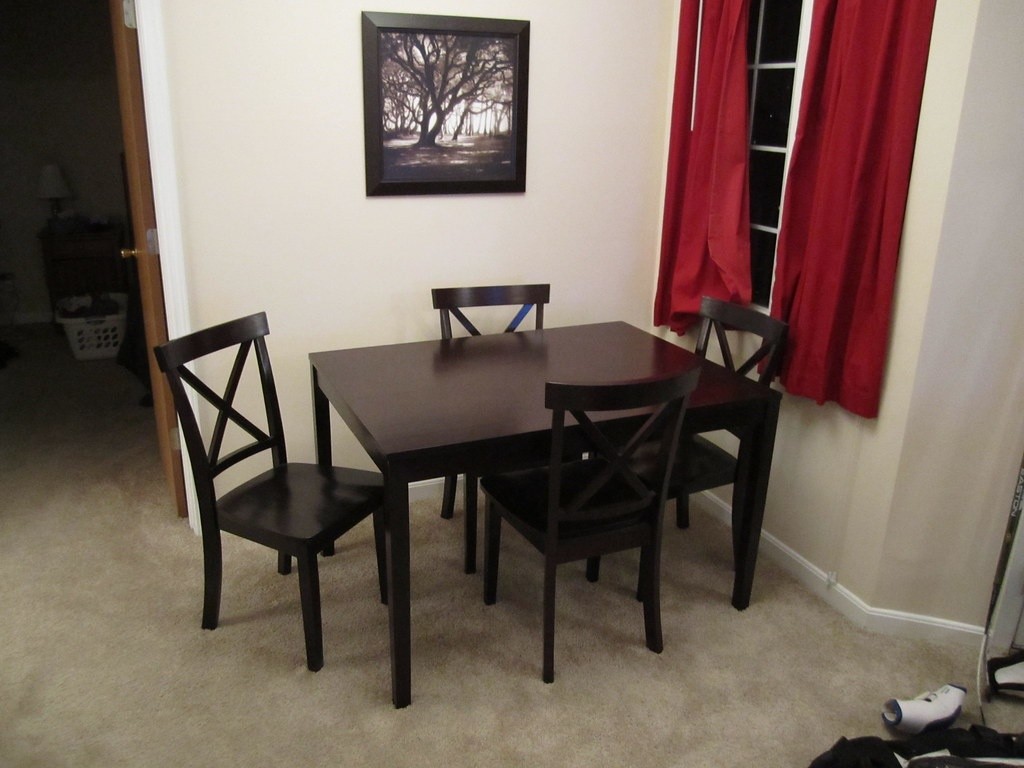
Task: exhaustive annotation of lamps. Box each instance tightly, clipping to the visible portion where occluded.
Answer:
[40,164,71,235]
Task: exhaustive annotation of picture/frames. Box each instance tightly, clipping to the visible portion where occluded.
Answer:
[362,11,529,197]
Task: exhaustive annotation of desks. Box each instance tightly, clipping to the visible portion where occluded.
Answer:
[308,320,783,709]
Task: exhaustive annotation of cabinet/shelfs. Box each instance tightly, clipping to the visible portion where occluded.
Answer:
[37,221,131,335]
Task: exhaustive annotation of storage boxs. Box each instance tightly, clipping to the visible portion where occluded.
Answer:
[55,293,128,360]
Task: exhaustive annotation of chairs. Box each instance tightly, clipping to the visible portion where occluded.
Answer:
[431,284,550,574]
[480,367,701,683]
[591,294,790,572]
[153,311,389,672]
